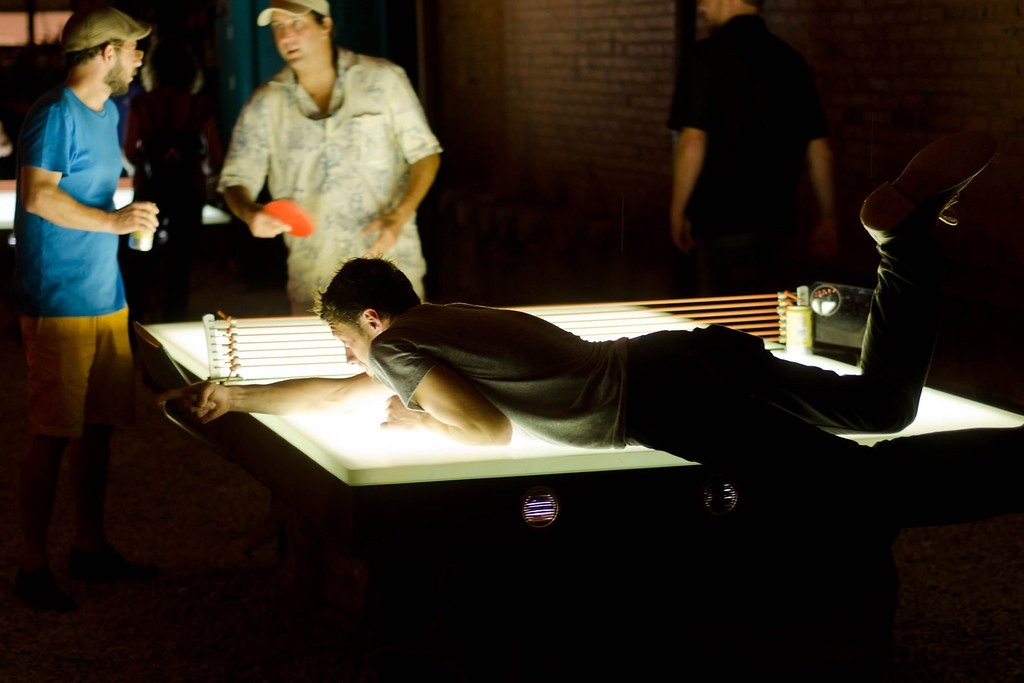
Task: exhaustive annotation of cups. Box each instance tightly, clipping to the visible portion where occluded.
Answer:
[126,225,156,250]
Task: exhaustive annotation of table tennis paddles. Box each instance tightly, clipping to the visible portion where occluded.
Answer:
[260,197,315,239]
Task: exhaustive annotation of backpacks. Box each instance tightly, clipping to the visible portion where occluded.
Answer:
[134,89,207,226]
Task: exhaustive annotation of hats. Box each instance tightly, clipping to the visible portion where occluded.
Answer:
[60,4,154,55]
[256,0,331,26]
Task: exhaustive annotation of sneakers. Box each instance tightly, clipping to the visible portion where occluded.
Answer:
[14,561,78,615]
[862,129,999,254]
[63,542,166,585]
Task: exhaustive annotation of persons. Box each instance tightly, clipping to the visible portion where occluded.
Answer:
[669,0,841,301]
[120,12,232,323]
[216,0,444,321]
[152,128,1023,534]
[11,5,162,618]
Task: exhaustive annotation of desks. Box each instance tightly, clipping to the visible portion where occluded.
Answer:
[134,302,1024,682]
